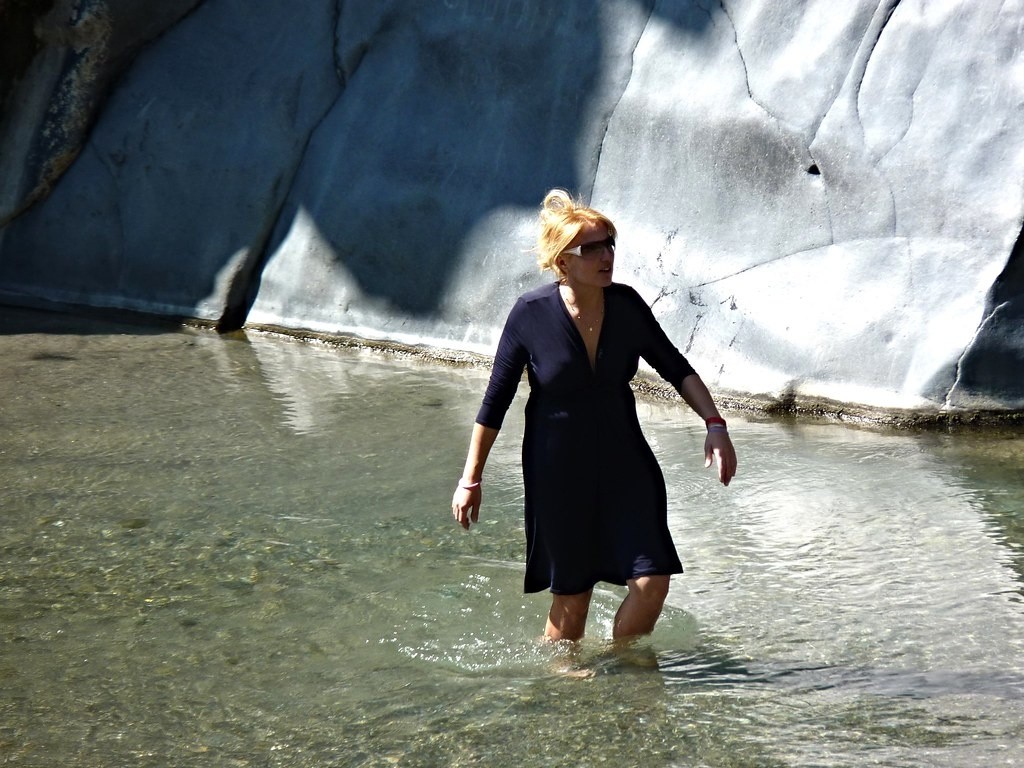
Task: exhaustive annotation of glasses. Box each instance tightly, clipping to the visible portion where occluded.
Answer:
[559,235,616,258]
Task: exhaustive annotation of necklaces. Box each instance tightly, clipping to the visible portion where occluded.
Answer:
[562,286,604,333]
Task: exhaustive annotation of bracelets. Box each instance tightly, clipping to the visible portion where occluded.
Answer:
[458,478,483,488]
[706,418,728,434]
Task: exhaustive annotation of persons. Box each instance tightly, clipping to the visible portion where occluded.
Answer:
[451,188,738,680]
